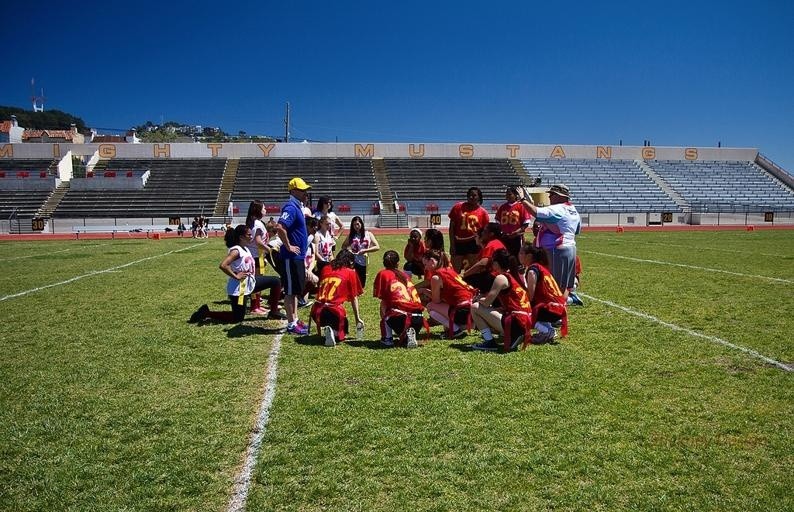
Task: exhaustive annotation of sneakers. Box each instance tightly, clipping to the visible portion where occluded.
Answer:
[285,324,307,335]
[324,326,336,346]
[296,319,309,329]
[379,324,553,352]
[569,292,583,306]
[250,296,312,319]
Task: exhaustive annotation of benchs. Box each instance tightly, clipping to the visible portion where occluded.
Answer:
[520,158,683,212]
[230,156,382,215]
[1,155,228,222]
[644,155,794,212]
[382,156,526,213]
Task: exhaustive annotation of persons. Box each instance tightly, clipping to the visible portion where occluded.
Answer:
[191,199,288,323]
[276,178,380,347]
[373,185,580,352]
[179,215,209,239]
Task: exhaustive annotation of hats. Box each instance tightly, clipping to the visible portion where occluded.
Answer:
[544,183,571,199]
[286,177,312,191]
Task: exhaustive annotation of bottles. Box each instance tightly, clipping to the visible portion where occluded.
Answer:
[356,322,364,342]
